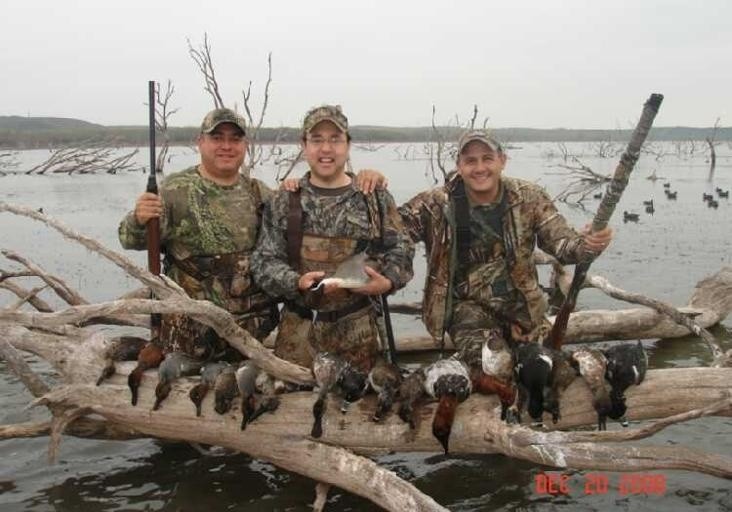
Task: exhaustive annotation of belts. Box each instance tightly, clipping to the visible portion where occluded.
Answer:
[287,300,370,321]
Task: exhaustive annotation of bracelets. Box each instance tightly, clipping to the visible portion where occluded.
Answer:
[134,211,143,228]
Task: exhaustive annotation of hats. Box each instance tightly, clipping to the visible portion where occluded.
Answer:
[201,108,246,137]
[458,131,502,153]
[305,107,348,133]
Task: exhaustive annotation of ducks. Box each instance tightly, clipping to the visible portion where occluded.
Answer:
[309,241,383,326]
[308,339,569,455]
[573,342,654,435]
[94,334,279,428]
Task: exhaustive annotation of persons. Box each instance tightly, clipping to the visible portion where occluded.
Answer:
[117,109,303,364]
[354,127,612,368]
[252,103,413,375]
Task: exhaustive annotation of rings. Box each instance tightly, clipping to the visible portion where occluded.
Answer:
[601,243,606,250]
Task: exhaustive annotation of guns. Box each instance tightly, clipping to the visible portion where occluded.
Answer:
[544,93,663,348]
[371,294,394,364]
[146,82,162,344]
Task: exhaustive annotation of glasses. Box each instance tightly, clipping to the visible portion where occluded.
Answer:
[305,137,347,148]
